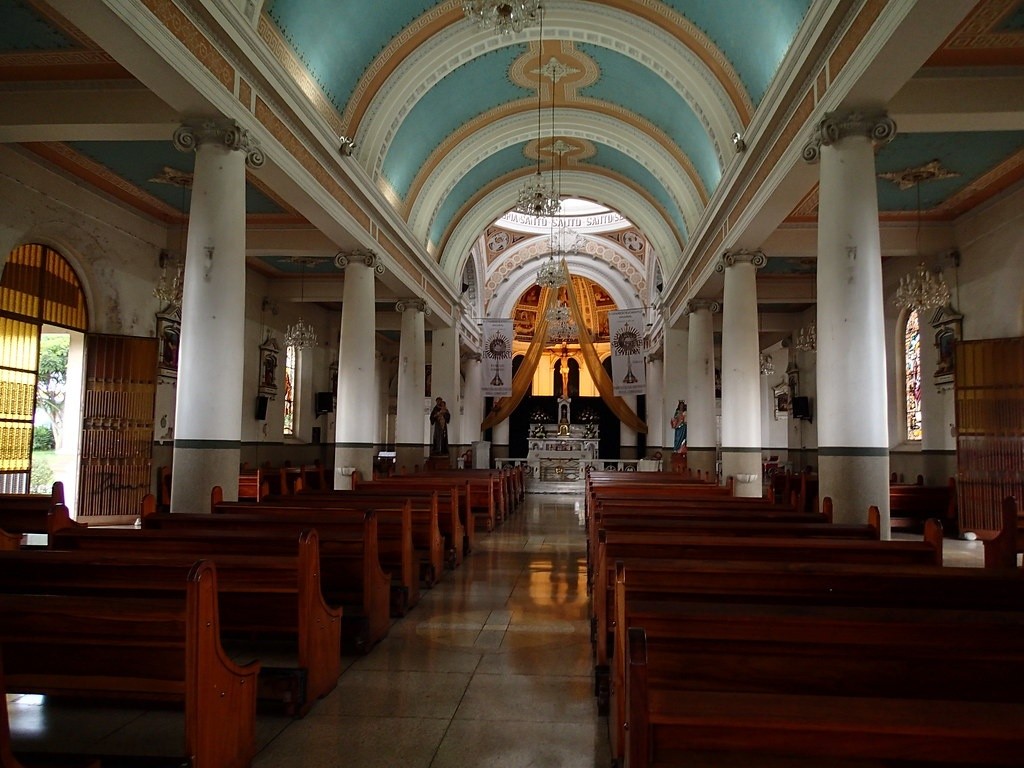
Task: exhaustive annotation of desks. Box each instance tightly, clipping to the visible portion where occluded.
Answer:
[585,464,1024,768]
[0,457,528,768]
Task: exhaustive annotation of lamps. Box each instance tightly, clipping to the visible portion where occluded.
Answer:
[464,0,607,341]
[878,160,959,314]
[147,163,195,308]
[759,299,775,376]
[282,257,319,353]
[795,264,817,353]
